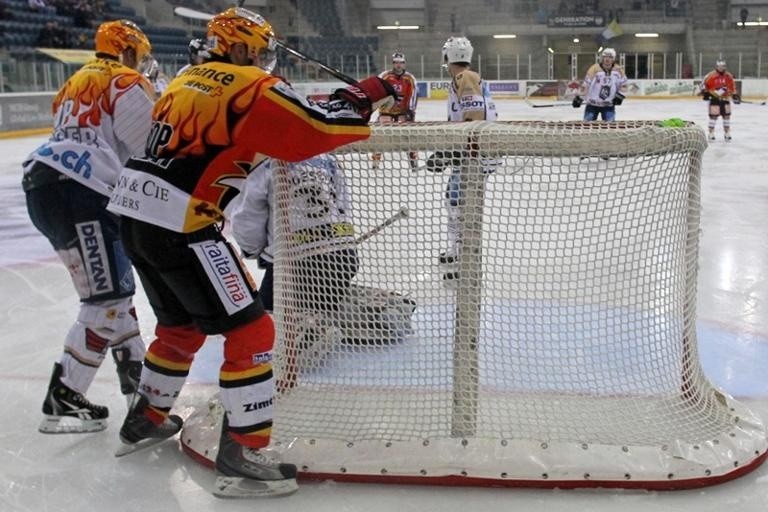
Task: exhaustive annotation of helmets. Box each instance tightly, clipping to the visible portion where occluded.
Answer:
[95,19,158,79]
[188,38,212,65]
[601,47,616,64]
[442,36,474,66]
[207,8,277,74]
[392,52,405,62]
[716,60,727,75]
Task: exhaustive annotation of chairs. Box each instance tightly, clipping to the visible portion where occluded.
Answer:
[1,0,381,92]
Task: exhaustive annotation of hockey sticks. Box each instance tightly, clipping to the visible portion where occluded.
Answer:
[174,7,359,87]
[524,97,588,109]
[710,97,768,105]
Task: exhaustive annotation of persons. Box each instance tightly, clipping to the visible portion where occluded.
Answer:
[427,35,505,285]
[695,60,742,142]
[572,47,628,161]
[370,50,420,173]
[231,148,419,370]
[19,21,165,434]
[0,1,107,92]
[111,5,404,498]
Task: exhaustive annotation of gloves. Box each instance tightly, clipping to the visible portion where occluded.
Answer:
[733,94,740,103]
[330,76,397,119]
[703,93,711,100]
[405,110,415,121]
[613,93,625,105]
[427,152,460,172]
[573,95,582,107]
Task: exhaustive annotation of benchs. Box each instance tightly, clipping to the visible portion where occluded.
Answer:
[378,0,766,85]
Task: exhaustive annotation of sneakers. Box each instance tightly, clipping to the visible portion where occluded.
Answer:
[120,411,182,444]
[42,381,108,420]
[219,434,296,479]
[117,362,143,394]
